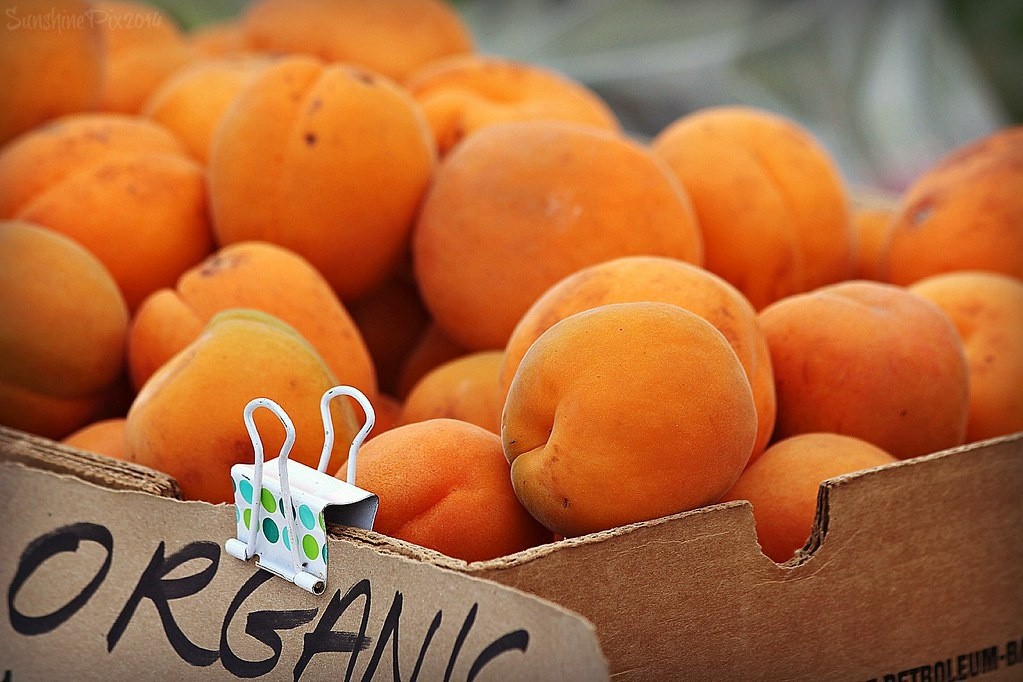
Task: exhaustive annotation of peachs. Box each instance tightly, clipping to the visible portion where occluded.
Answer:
[0,0,1023,570]
